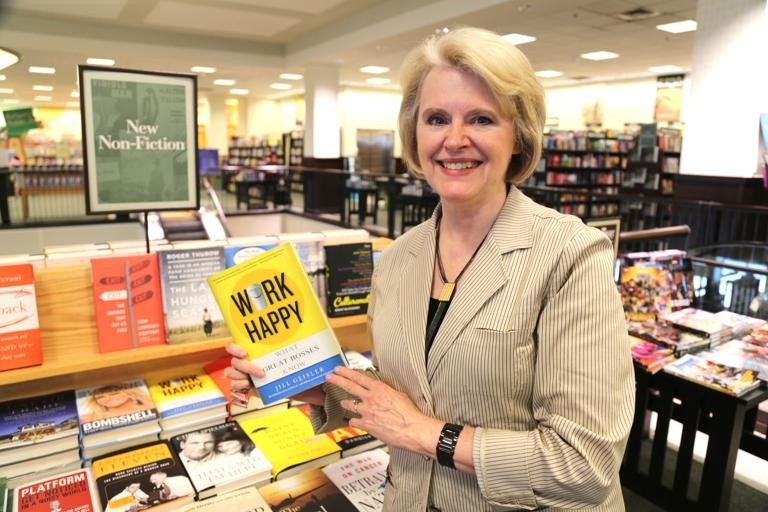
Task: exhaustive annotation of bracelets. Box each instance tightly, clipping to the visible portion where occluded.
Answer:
[435,421,463,471]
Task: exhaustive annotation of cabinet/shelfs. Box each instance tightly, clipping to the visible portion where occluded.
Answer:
[225,131,299,198]
[532,127,645,231]
[0,307,391,512]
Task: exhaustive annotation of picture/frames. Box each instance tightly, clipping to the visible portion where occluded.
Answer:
[77,61,202,215]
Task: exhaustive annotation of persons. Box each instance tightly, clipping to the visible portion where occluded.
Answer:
[105,471,194,511]
[79,383,158,446]
[223,24,639,511]
[0,144,16,230]
[178,429,270,492]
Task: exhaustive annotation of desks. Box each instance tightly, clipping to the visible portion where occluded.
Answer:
[618,301,767,512]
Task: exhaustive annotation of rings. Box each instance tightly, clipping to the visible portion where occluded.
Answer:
[352,400,359,414]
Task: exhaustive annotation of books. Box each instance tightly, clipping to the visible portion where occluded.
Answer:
[203,347,394,511]
[208,242,349,408]
[610,246,768,401]
[0,379,196,511]
[158,246,231,344]
[513,126,679,233]
[225,229,374,318]
[1,240,229,264]
[1,264,42,371]
[146,367,273,512]
[90,254,165,354]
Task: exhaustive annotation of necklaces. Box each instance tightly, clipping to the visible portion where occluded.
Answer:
[433,227,491,303]
